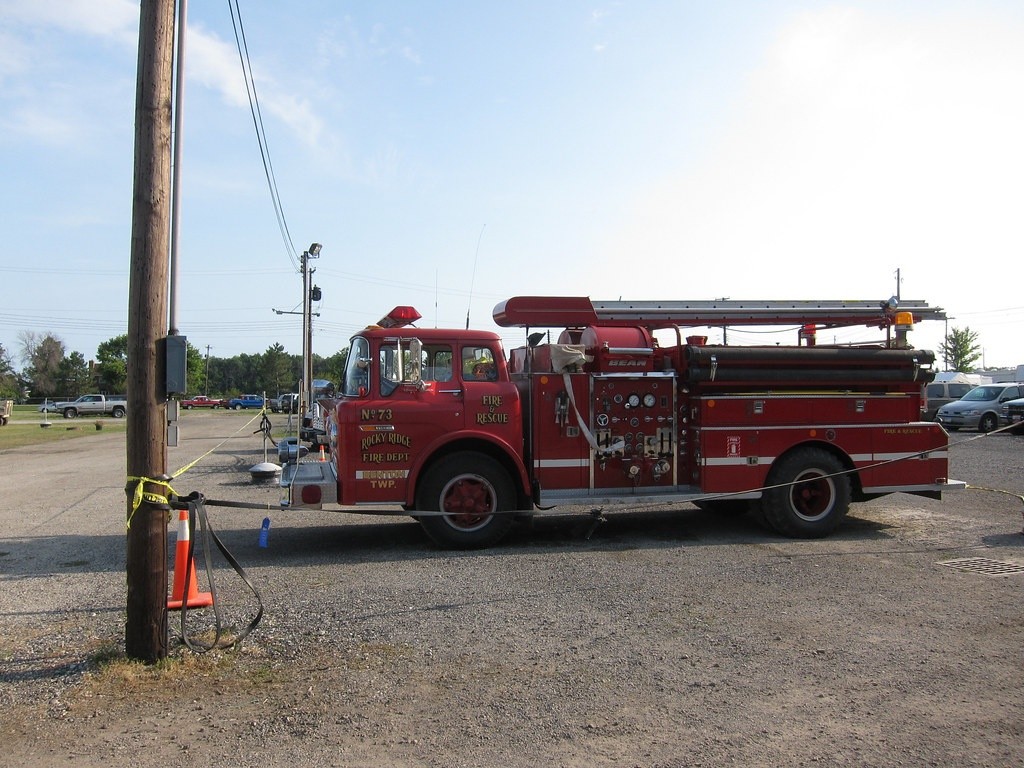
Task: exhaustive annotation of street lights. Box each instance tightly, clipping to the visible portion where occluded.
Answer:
[305,242,319,428]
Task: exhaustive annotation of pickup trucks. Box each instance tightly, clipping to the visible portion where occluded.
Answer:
[56,394,127,418]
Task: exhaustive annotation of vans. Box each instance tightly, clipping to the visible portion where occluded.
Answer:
[932,381,1024,433]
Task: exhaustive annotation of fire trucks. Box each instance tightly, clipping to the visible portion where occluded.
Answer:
[285,298,968,551]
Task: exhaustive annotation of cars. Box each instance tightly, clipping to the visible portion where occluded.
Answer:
[269,394,298,413]
[920,381,978,422]
[299,398,336,443]
[1002,399,1024,435]
[289,394,330,415]
[227,394,263,411]
[39,402,61,413]
[180,396,223,409]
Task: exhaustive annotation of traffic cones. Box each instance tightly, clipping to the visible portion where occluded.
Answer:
[318,445,326,461]
[165,510,212,608]
